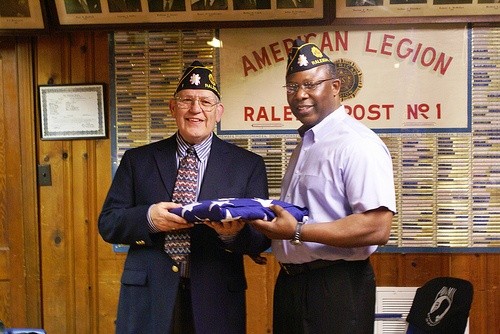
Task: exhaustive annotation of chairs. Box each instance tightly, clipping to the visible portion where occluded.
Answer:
[374,277,473,334]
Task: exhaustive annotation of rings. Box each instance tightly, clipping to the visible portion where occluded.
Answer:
[172,228,176,231]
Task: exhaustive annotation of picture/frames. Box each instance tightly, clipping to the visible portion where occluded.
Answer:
[0,0,51,37]
[327,0,500,26]
[43,0,330,34]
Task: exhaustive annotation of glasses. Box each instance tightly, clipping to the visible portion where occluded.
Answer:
[175,97,220,112]
[283,78,334,95]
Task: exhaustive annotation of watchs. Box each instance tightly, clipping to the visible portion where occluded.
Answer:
[290,223,304,246]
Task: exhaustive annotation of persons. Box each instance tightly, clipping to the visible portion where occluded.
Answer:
[246,39,397,334]
[98,61,272,334]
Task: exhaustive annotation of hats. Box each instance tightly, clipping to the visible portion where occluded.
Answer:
[174,60,221,98]
[285,38,334,74]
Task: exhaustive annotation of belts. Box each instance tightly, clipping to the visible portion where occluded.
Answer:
[279,259,344,275]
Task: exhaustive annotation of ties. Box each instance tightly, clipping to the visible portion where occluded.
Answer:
[163,147,199,264]
